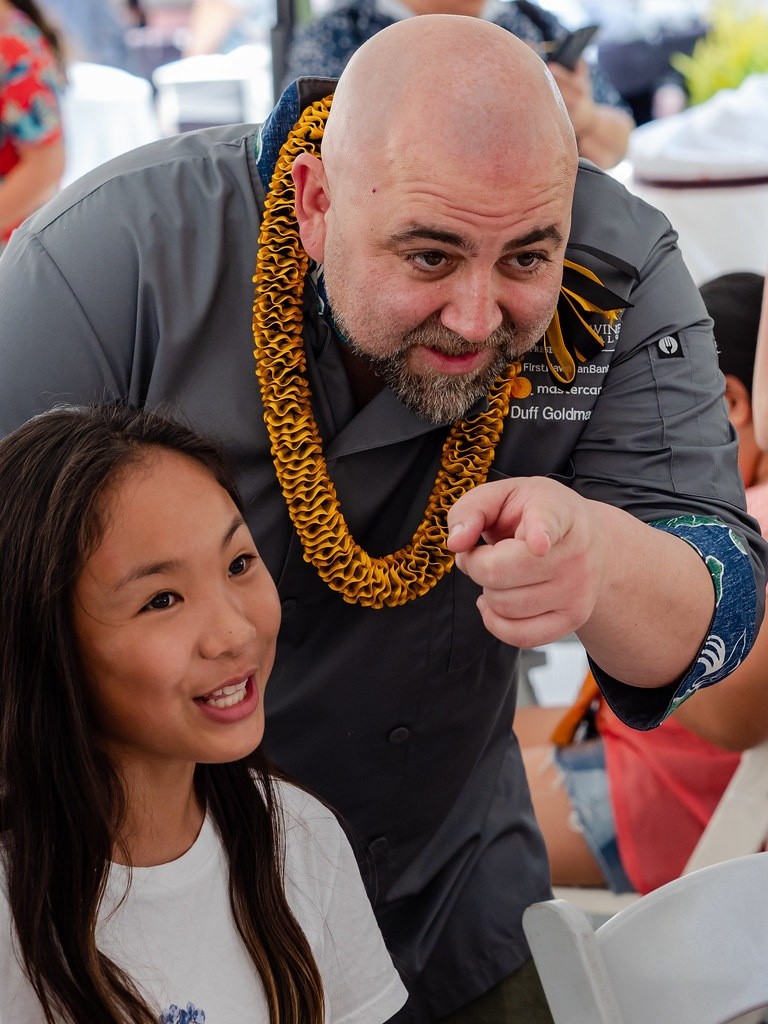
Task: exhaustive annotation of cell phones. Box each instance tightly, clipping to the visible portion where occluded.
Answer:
[547,24,601,71]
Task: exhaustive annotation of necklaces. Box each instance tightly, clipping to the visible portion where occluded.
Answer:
[252,94,521,607]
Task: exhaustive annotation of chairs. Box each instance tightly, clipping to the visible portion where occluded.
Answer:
[522,847,768,1024]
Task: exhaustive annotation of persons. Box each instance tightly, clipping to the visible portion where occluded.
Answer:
[271,0,632,167]
[0,13,768,1024]
[0,0,68,253]
[0,406,408,1024]
[515,273,768,886]
